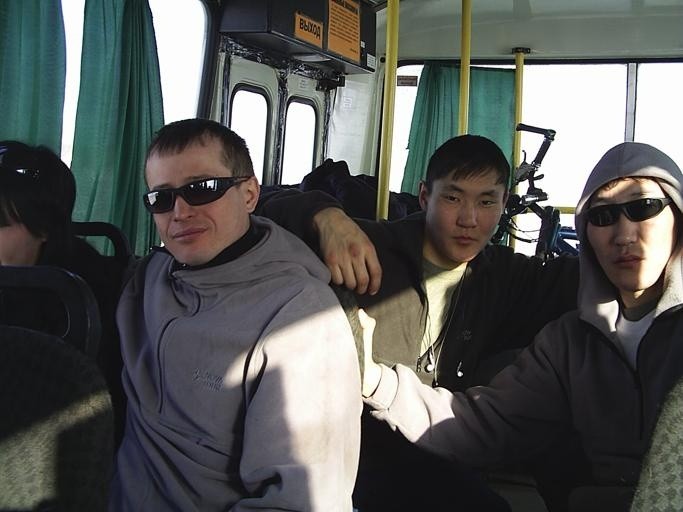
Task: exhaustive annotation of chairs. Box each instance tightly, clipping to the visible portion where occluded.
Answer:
[0,265,122,512]
[67,218,135,277]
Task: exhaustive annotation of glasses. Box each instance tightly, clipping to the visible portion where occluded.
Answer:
[0,163,44,183]
[584,197,674,227]
[141,176,251,215]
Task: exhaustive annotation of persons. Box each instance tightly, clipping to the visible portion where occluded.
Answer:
[358,143,682,511]
[250,134,582,512]
[100,118,362,512]
[0,139,129,512]
